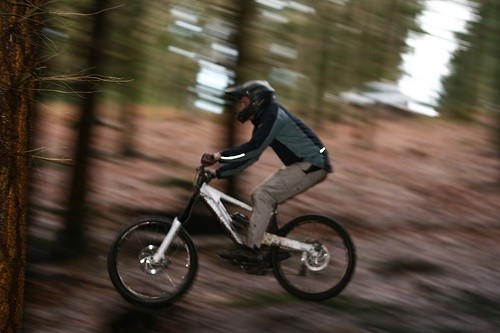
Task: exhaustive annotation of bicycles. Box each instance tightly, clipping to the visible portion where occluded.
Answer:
[107,151,358,308]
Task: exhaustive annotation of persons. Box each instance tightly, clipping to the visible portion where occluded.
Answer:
[201,81,333,262]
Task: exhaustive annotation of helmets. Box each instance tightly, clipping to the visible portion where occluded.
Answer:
[225,79,278,124]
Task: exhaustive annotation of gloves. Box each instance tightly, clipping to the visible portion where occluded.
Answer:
[204,169,217,183]
[200,152,215,165]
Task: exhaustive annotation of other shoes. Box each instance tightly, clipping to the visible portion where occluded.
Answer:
[218,245,265,266]
[266,246,291,263]
